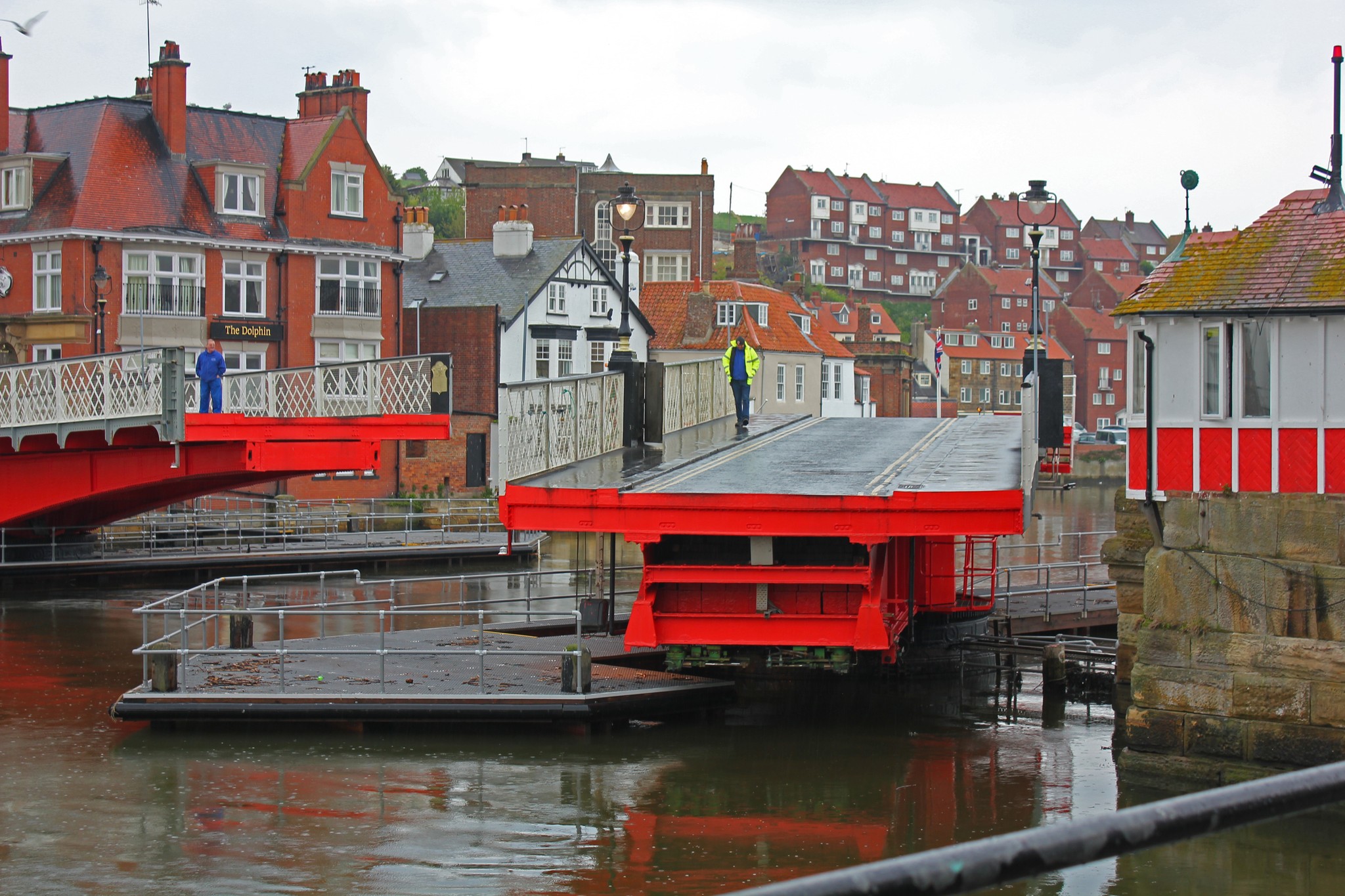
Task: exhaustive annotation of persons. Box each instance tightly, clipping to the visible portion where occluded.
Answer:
[722,336,761,426]
[195,339,226,414]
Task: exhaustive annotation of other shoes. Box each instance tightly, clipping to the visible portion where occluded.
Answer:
[735,422,742,427]
[743,418,749,426]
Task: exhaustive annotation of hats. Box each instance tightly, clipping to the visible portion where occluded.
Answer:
[736,337,744,345]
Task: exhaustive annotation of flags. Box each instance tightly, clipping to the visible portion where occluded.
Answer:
[934,329,942,377]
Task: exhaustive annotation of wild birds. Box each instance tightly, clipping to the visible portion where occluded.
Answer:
[0,10,48,36]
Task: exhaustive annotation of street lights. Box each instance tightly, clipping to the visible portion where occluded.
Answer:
[89,265,114,412]
[1016,179,1057,381]
[607,181,646,447]
